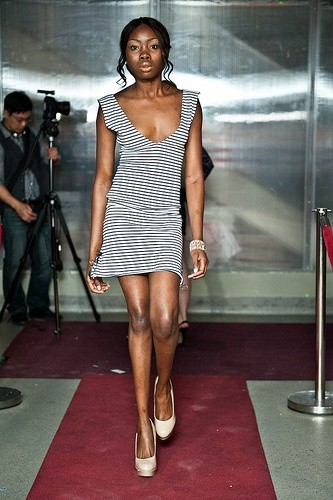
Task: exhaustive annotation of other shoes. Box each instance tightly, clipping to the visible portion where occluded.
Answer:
[10,312,29,326]
[0,387,24,408]
[29,307,63,321]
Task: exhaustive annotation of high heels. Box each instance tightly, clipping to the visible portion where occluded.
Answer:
[177,321,191,345]
[134,418,157,477]
[153,375,176,441]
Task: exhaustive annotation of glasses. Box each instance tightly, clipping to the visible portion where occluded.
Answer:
[11,116,32,122]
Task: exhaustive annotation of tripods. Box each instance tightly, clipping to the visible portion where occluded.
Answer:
[0,118,101,339]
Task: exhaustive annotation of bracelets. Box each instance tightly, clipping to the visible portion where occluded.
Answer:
[189,241,204,250]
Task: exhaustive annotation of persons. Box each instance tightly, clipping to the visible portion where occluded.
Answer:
[0,90,61,324]
[85,19,214,477]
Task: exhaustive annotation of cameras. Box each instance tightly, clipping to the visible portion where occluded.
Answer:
[43,96,70,118]
